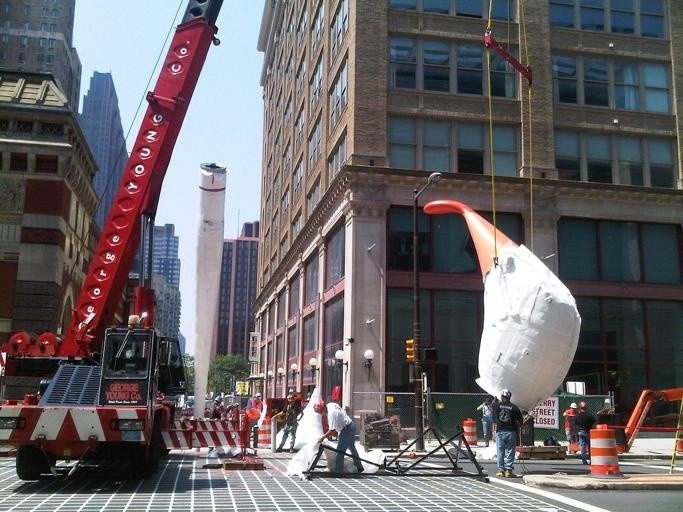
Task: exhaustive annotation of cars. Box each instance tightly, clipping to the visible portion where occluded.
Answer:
[177,399,216,419]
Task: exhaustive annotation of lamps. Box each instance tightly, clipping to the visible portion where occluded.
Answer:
[363,349,374,381]
[259,350,349,389]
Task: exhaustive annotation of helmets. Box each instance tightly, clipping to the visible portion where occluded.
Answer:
[579,400,587,408]
[287,394,294,399]
[570,402,577,408]
[314,400,325,414]
[501,389,511,397]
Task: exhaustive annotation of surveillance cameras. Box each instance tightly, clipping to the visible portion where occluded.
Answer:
[366,319,375,325]
[346,340,349,346]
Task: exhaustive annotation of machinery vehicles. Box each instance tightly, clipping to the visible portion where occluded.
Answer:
[0,1,264,484]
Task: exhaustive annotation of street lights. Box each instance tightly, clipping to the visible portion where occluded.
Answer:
[409,171,443,451]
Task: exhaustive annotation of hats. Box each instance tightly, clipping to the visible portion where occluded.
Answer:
[255,392,262,401]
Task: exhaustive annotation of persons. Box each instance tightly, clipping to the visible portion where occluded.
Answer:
[204,396,240,431]
[493,389,523,478]
[246,393,263,448]
[271,395,304,452]
[562,403,581,454]
[476,396,497,447]
[314,400,364,474]
[575,401,596,465]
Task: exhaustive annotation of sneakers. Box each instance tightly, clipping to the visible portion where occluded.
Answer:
[581,458,589,465]
[289,446,294,453]
[358,468,365,473]
[277,447,283,453]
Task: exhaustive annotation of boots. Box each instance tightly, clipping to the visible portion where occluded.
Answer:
[496,469,505,477]
[505,470,517,478]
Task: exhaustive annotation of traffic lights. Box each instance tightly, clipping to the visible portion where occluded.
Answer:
[406,339,414,362]
[422,347,439,364]
